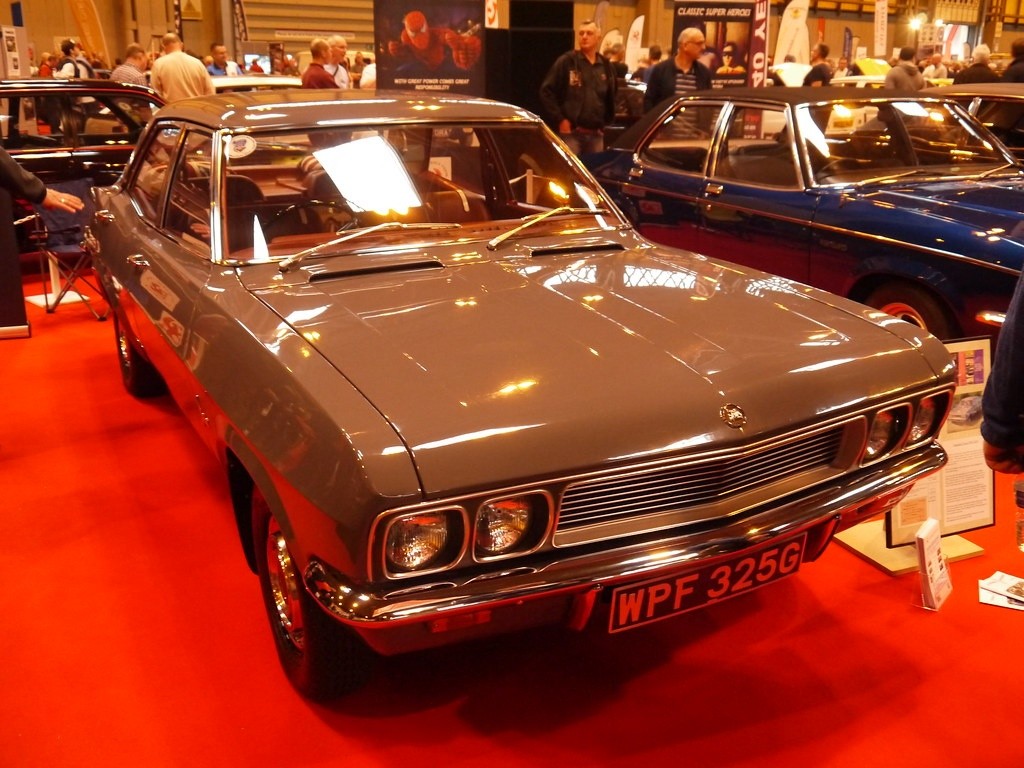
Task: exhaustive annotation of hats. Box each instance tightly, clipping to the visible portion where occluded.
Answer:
[61,39,75,52]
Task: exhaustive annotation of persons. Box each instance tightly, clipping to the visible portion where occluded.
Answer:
[150,33,216,103]
[206,42,244,76]
[643,27,714,140]
[921,53,947,78]
[541,21,628,156]
[979,267,1024,474]
[716,42,745,74]
[885,46,925,91]
[40,38,110,134]
[803,43,833,132]
[952,38,1024,84]
[249,59,264,73]
[111,42,153,122]
[0,146,85,213]
[833,57,848,78]
[301,35,376,89]
[774,54,796,86]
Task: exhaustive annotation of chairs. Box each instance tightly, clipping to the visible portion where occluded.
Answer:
[185,168,490,221]
[651,141,906,186]
[36,178,110,320]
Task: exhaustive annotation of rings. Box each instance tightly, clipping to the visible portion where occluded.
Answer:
[61,198,64,203]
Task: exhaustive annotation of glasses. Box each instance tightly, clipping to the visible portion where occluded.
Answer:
[723,51,734,57]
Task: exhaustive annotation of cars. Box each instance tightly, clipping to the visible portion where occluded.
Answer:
[0,80,170,252]
[768,57,1024,160]
[89,87,956,707]
[580,88,1024,344]
